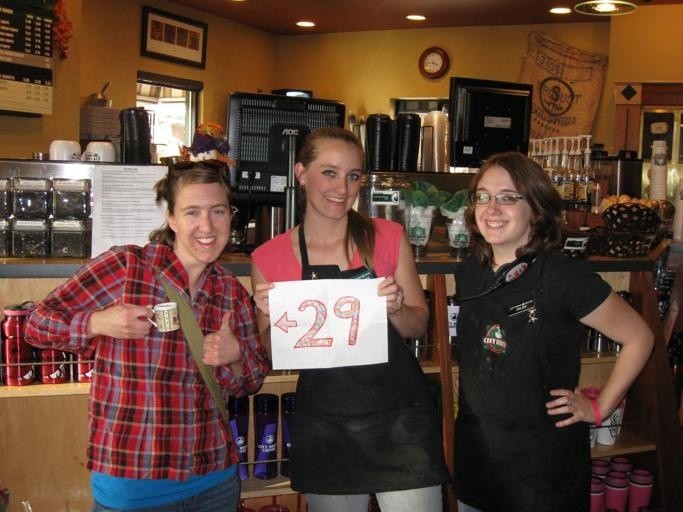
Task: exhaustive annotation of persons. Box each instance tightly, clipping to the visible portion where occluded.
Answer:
[250,127,451,511]
[25,157,268,511]
[422,151,656,512]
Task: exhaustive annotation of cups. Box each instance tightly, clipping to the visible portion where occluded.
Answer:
[365,107,451,173]
[145,303,183,333]
[648,140,683,240]
[119,107,155,164]
[584,387,626,447]
[48,138,81,161]
[81,141,118,163]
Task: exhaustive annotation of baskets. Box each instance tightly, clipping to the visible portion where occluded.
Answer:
[582,225,660,258]
[600,202,666,234]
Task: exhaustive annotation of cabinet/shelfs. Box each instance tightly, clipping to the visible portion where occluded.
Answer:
[226,260,306,512]
[580,252,671,507]
[407,257,457,511]
[1,259,91,511]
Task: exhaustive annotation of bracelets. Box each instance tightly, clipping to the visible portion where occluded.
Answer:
[591,399,602,427]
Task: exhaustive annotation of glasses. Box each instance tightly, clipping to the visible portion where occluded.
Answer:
[470,192,524,205]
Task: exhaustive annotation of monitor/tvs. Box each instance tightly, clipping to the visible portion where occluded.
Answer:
[226,91,345,202]
[447,77,532,168]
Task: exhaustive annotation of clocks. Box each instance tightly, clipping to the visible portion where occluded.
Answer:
[417,46,450,81]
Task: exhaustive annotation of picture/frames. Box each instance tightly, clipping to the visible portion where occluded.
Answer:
[138,3,208,70]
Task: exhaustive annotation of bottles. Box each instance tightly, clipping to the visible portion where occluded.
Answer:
[530,136,592,211]
[229,395,295,480]
[588,455,653,511]
[590,286,631,354]
[448,286,459,356]
[3,305,96,385]
